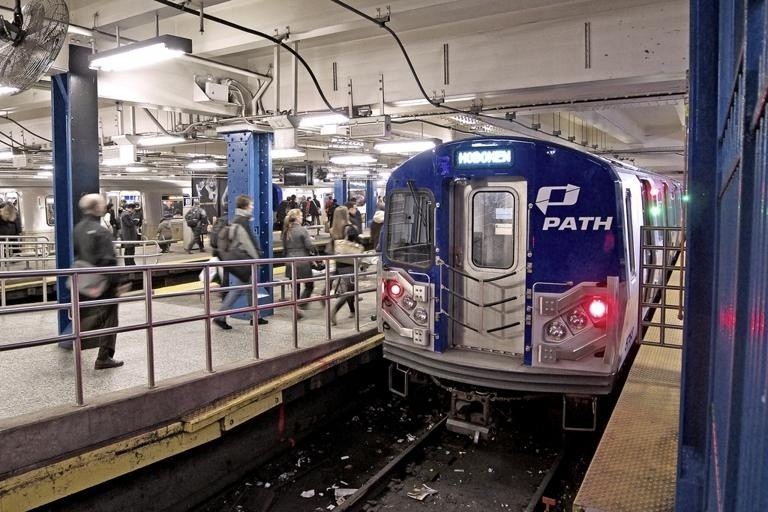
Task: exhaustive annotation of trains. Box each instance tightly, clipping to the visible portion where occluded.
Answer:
[0,176,192,255]
[374,136,686,446]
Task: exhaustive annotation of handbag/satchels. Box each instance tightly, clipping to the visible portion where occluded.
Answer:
[66,261,109,298]
[309,252,325,271]
[222,247,255,282]
[334,239,365,265]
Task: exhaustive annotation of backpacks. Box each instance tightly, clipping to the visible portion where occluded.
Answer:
[187,207,201,227]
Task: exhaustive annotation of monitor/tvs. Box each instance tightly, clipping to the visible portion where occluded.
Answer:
[307,165,314,186]
[284,164,308,186]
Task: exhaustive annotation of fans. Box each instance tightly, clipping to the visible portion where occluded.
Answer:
[0,0,69,97]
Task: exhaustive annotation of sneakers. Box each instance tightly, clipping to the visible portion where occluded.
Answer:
[331,309,337,326]
[291,305,303,318]
[349,311,355,317]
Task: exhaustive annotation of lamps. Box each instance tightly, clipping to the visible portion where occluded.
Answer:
[298,113,349,126]
[126,167,149,172]
[271,149,305,158]
[373,121,443,153]
[85,14,192,73]
[329,154,378,164]
[185,144,227,168]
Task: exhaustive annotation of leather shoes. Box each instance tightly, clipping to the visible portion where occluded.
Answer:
[213,318,232,329]
[94,357,124,370]
[251,317,268,325]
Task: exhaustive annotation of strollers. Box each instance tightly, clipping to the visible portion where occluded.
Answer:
[355,246,373,273]
